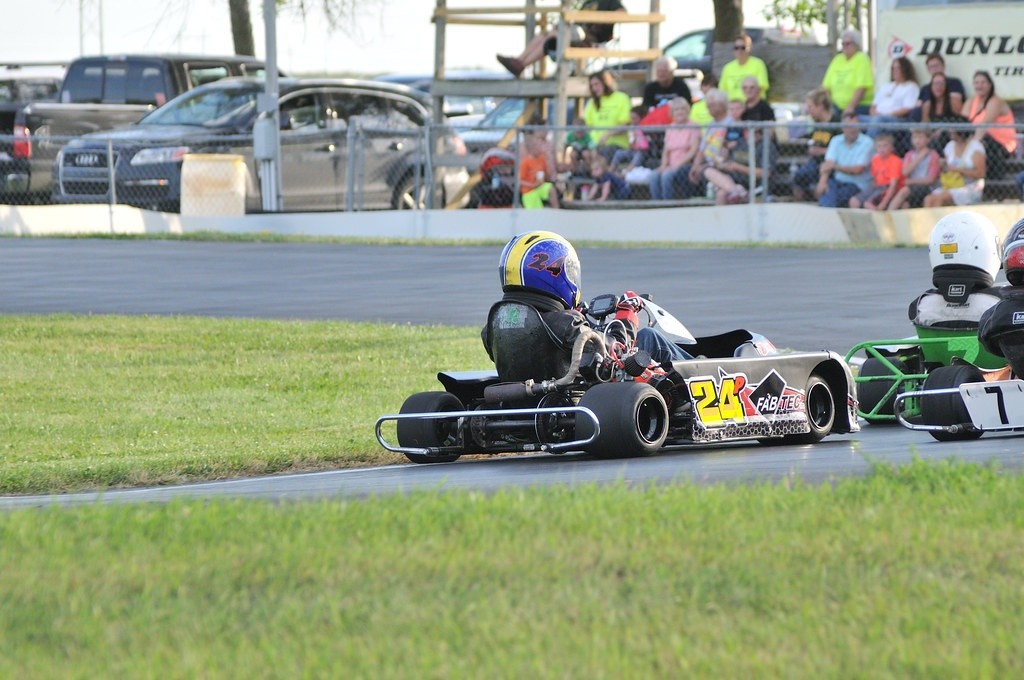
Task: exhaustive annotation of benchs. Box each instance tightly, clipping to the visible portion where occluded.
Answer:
[563,105,1024,201]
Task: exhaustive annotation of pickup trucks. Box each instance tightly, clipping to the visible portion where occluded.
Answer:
[12,51,291,177]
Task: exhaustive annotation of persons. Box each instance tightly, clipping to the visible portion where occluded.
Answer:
[520,71,781,207]
[643,57,691,106]
[922,73,962,154]
[813,111,875,206]
[913,55,966,121]
[925,117,987,208]
[908,212,1002,330]
[962,69,1017,158]
[718,35,769,102]
[794,88,840,201]
[821,28,874,121]
[849,133,905,208]
[976,218,1024,377]
[420,134,477,208]
[858,58,920,137]
[495,0,627,77]
[888,129,940,209]
[480,232,707,382]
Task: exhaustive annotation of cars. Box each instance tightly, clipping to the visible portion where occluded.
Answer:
[379,28,763,165]
[0,76,65,135]
[0,134,33,206]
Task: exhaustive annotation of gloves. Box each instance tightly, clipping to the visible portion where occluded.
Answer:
[617,290,645,314]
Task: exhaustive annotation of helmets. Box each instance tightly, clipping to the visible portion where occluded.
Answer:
[480,148,517,188]
[1003,218,1024,286]
[498,229,582,310]
[927,209,1003,281]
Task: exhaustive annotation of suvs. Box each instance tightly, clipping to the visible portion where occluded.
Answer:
[51,73,473,215]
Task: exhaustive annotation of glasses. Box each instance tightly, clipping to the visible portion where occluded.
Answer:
[842,41,852,45]
[734,45,746,50]
